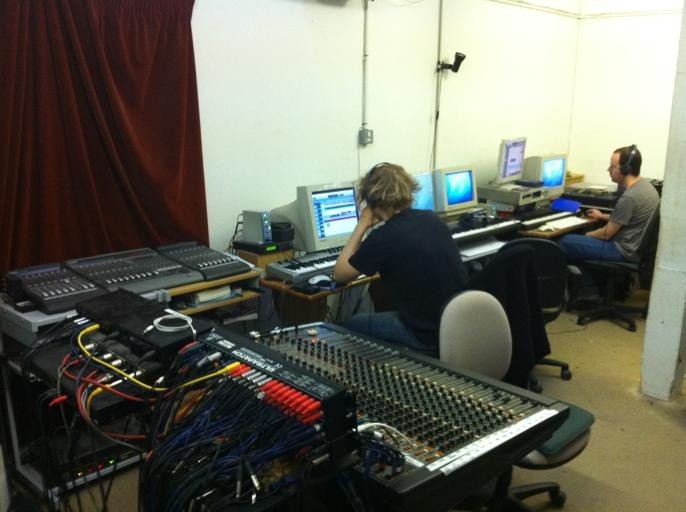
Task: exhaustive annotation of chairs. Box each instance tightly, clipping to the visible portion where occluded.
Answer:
[572,201,661,331]
[437,289,595,512]
[465,243,571,394]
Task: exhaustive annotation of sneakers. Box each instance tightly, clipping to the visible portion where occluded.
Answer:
[569,285,599,304]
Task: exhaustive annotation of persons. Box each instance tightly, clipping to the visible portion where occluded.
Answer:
[333,162,469,359]
[561,145,661,311]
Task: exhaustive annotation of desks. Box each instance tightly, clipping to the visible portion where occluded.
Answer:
[236,208,600,326]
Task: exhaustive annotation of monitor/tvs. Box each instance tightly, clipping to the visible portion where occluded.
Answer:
[268,181,362,253]
[411,173,436,214]
[495,136,527,190]
[522,153,568,199]
[431,163,479,226]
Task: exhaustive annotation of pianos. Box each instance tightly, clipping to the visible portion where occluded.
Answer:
[266,247,344,283]
[513,208,581,230]
[446,216,521,246]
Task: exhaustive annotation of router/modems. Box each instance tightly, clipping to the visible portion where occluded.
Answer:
[242,208,272,244]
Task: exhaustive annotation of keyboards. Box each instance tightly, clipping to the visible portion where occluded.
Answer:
[458,240,507,259]
[546,215,590,231]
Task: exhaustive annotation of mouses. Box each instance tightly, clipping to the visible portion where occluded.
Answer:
[308,274,332,286]
[538,224,554,232]
[486,235,496,241]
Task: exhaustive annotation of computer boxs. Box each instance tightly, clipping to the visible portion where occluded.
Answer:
[476,183,550,206]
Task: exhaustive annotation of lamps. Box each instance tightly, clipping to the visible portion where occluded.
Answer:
[438,51,466,73]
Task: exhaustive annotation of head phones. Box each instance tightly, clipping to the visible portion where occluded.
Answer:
[620,144,637,176]
[363,162,390,210]
